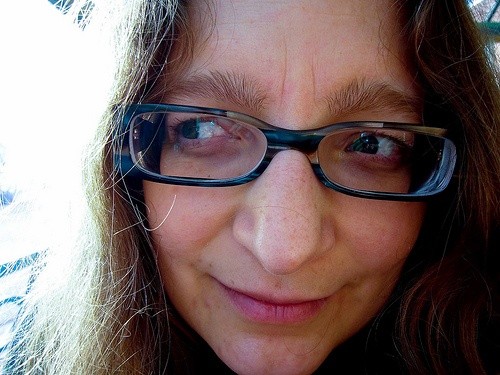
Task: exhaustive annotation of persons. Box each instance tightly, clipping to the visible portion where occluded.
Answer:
[0,0,500,375]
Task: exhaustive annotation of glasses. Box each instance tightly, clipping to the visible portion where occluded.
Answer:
[126,104,463,202]
